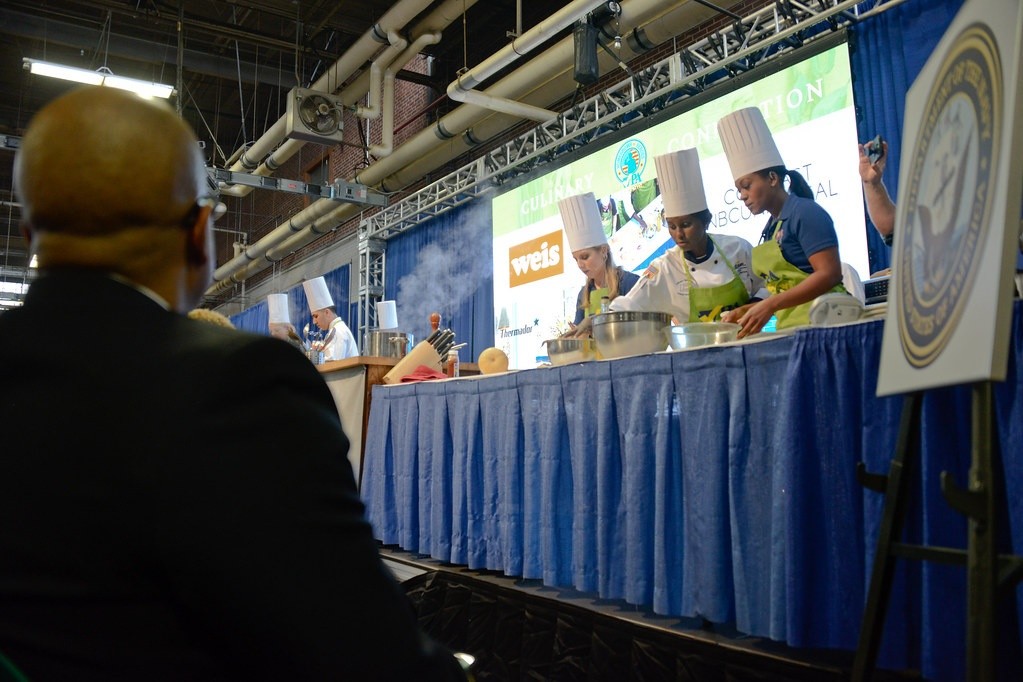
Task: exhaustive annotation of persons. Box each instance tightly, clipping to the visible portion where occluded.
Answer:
[303,276,359,361]
[557,192,639,339]
[558,148,772,346]
[715,106,850,338]
[859,140,896,246]
[268,293,304,352]
[0,86,469,682]
[596,179,658,237]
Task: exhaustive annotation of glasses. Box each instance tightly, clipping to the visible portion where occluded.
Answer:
[184,196,227,230]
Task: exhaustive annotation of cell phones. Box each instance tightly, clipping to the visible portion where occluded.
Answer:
[866,136,884,164]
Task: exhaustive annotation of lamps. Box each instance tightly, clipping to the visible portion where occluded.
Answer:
[20,11,175,99]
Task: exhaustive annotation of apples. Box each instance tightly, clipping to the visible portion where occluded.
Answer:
[478,347,509,374]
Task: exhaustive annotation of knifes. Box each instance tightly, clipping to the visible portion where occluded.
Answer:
[426,328,456,357]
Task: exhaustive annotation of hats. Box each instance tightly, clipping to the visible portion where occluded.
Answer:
[267,293,290,324]
[655,147,709,217]
[303,276,334,312]
[718,105,784,182]
[558,192,607,253]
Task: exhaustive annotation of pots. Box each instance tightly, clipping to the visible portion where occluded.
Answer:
[363,331,408,359]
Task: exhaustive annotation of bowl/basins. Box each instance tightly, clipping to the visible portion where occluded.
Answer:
[660,321,743,351]
[544,339,598,366]
[591,311,673,358]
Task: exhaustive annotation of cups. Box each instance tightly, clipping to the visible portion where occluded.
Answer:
[310,351,324,365]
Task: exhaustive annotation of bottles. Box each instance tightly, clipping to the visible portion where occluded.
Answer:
[447,350,460,377]
[600,295,609,313]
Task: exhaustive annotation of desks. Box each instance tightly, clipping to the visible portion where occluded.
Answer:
[358,296,1022,682]
